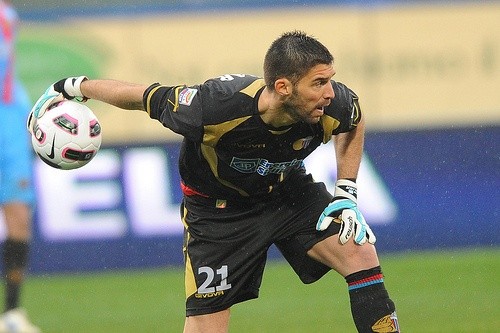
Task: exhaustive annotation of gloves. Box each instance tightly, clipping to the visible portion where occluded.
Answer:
[316,179,376,246]
[27,75,91,135]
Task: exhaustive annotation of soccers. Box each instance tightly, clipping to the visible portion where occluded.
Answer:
[31,98,102,172]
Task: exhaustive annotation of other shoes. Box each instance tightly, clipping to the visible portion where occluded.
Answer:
[0,310,40,333]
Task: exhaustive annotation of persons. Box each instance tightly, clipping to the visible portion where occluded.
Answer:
[0,0,43,333]
[34,32,401,333]
